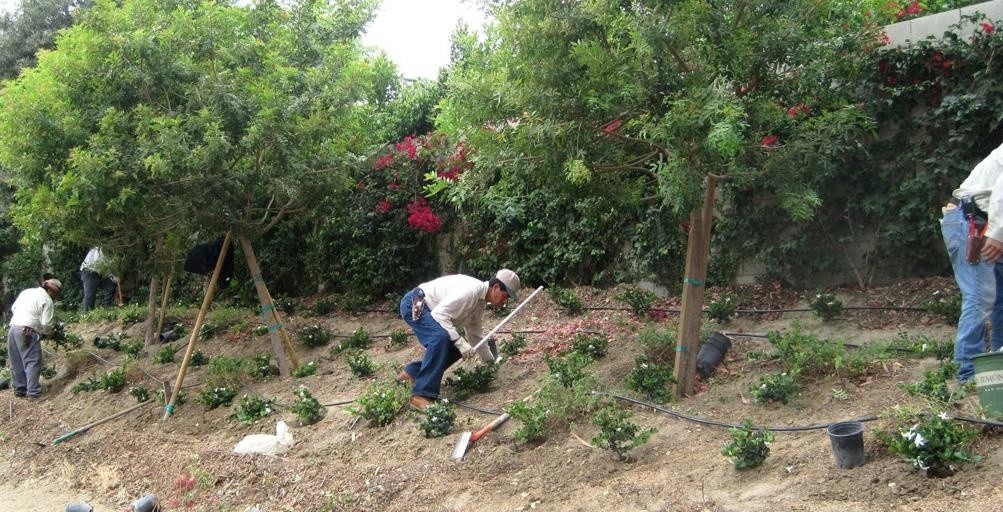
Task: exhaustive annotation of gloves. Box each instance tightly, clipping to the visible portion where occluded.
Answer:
[478,348,494,361]
[454,335,474,359]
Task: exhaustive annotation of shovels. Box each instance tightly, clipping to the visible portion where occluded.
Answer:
[32,397,156,449]
[449,378,555,461]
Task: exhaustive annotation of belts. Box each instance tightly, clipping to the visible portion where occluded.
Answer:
[950,196,987,223]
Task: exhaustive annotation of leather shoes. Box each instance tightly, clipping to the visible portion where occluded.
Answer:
[398,369,435,411]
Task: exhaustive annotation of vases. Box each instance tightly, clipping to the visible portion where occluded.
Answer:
[160,330,179,343]
[698,329,731,377]
[827,420,867,463]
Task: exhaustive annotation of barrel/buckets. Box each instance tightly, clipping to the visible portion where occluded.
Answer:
[697,332,731,375]
[828,421,865,468]
[970,351,1003,426]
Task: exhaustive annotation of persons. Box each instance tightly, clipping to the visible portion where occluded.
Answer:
[8,278,62,398]
[79,245,124,312]
[941,143,1003,381]
[397,269,520,411]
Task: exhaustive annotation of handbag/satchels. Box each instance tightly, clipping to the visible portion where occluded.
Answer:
[24,328,33,336]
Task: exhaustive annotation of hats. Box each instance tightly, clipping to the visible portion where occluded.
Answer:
[496,269,521,298]
[44,279,63,293]
